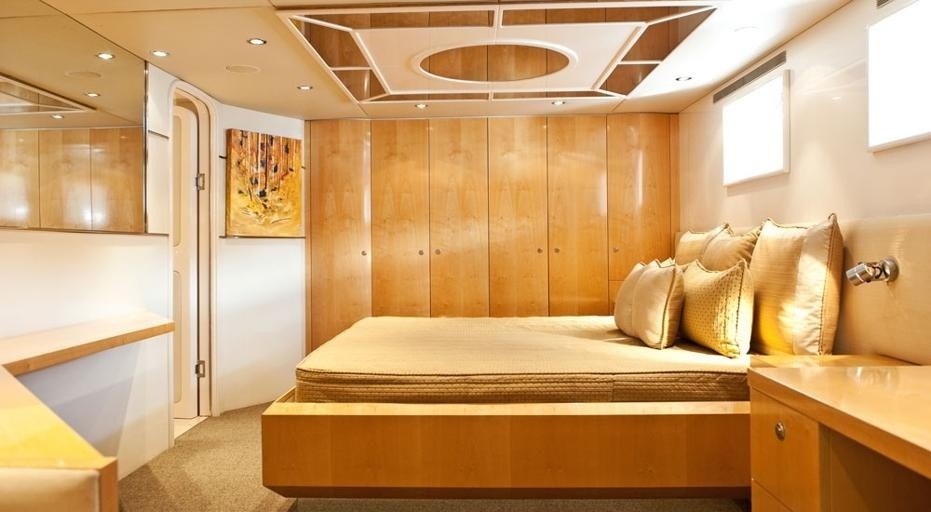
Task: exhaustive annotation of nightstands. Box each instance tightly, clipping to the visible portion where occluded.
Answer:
[746,363,929,512]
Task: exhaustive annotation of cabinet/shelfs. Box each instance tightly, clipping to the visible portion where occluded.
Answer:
[611,114,675,318]
[372,116,490,317]
[490,113,609,318]
[305,118,372,353]
[2,128,146,235]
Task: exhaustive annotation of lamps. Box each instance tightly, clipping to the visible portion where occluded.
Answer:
[845,254,897,285]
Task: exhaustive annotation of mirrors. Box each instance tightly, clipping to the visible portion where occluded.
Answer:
[0,0,148,235]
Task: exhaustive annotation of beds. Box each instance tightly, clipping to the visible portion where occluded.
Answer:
[259,213,929,500]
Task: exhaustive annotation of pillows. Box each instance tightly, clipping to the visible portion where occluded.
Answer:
[613,215,845,360]
